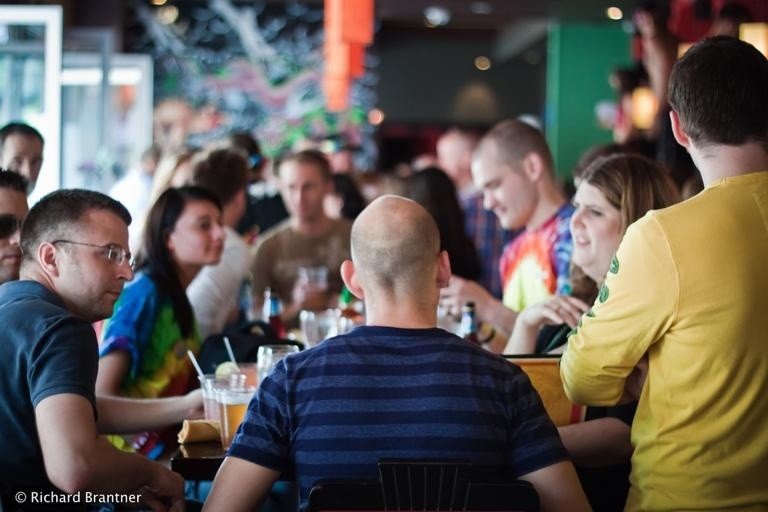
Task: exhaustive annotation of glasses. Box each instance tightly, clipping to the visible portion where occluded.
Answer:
[51,239,130,265]
[1,214,20,239]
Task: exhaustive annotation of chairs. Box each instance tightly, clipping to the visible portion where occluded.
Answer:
[299,460,542,512]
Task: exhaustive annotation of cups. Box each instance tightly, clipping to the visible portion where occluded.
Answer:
[197,263,363,457]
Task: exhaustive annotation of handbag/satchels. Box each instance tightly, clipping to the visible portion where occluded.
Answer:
[200,321,305,375]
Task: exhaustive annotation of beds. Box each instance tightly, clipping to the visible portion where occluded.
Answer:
[173,439,224,482]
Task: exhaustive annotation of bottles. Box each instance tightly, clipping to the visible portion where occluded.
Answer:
[459,301,482,349]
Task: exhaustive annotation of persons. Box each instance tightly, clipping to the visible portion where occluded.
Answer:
[0,121,45,197]
[198,191,596,511]
[0,169,30,283]
[554,32,768,511]
[92,114,708,502]
[0,185,191,511]
